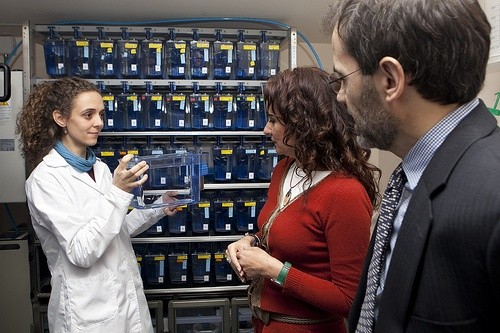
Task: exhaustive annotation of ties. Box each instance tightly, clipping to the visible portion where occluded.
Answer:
[354,162,408,333]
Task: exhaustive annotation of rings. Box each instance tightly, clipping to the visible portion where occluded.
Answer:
[226,257,232,263]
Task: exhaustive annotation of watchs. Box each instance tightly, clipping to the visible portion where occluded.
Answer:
[271,261,292,287]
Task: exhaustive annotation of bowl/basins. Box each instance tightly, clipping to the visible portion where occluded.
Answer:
[117,152,202,209]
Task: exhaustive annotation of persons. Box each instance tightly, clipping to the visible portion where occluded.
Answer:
[224,66,382,333]
[14,77,183,333]
[320,0,500,333]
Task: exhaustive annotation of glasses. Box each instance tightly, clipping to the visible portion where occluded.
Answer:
[329,68,361,94]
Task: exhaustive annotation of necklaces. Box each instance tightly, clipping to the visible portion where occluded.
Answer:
[283,165,309,205]
